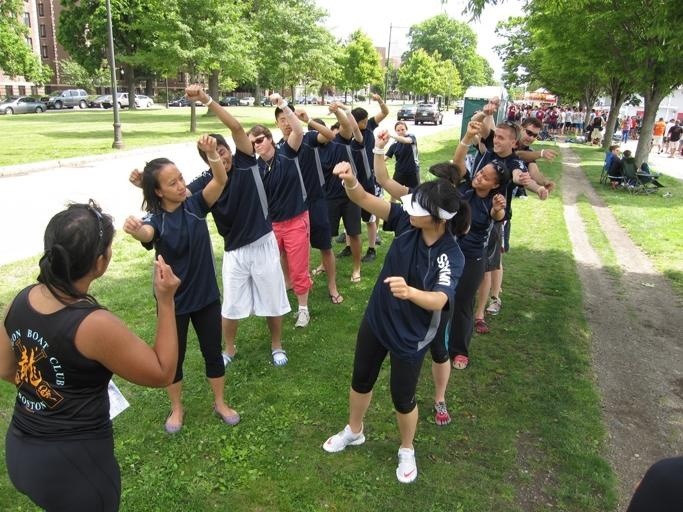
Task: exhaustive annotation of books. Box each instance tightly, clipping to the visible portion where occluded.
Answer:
[105,378,129,421]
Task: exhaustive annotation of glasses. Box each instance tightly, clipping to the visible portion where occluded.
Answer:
[250,136,266,147]
[71,203,104,252]
[491,157,506,179]
[525,128,538,138]
[502,119,518,139]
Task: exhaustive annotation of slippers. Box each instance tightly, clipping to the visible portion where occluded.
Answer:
[311,268,325,276]
[328,292,344,305]
[350,273,361,283]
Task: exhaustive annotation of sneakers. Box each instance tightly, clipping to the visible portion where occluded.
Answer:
[452,353,469,370]
[321,422,366,454]
[271,346,289,368]
[485,295,502,316]
[213,403,241,427]
[375,231,382,245]
[433,399,452,426]
[335,232,346,243]
[361,249,377,263]
[221,344,239,370]
[164,409,186,436]
[294,308,312,329]
[474,316,489,335]
[336,246,352,258]
[395,443,419,485]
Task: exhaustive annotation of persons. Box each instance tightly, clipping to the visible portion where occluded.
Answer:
[620,151,650,183]
[124,83,422,432]
[0,199,180,512]
[322,102,559,484]
[507,97,683,157]
[604,145,623,177]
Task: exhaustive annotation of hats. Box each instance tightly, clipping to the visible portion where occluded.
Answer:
[399,190,458,221]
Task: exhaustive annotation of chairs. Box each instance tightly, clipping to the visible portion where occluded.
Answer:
[600,150,659,195]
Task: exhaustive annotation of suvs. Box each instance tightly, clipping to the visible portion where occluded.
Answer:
[414,104,443,125]
[39,89,90,110]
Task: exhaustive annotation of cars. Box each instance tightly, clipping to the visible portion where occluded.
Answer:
[0,96,47,115]
[396,104,416,120]
[90,93,153,110]
[453,103,463,114]
[168,93,358,107]
